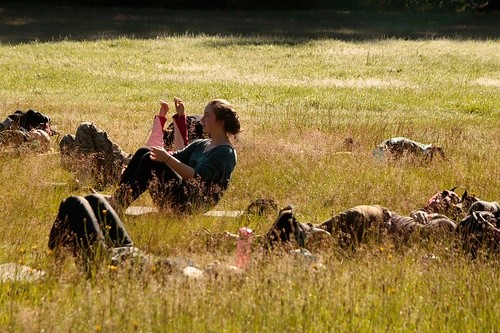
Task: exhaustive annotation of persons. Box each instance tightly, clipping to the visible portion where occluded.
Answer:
[81,100,241,221]
[285,226,333,283]
[47,189,251,298]
[265,204,457,258]
[59,97,206,182]
